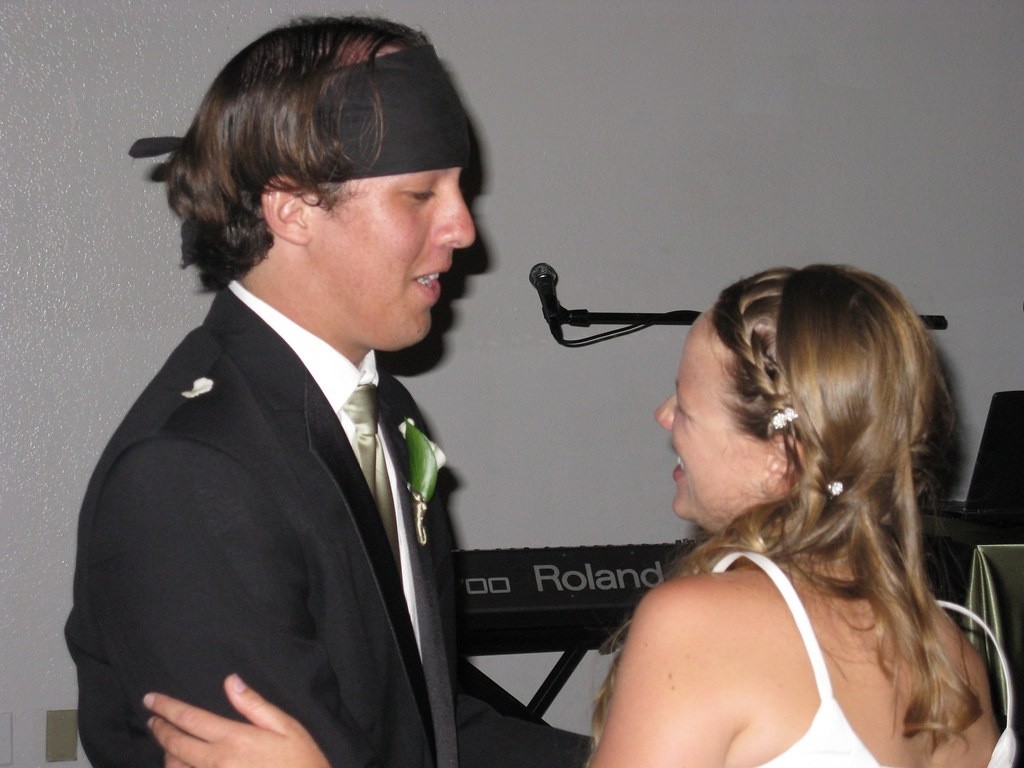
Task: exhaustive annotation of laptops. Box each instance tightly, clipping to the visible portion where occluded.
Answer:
[921,390,1024,521]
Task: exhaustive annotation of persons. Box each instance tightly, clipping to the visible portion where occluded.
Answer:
[66,15,594,768]
[144,265,1016,768]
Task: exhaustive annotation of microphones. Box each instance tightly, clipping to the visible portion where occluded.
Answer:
[529,262,563,344]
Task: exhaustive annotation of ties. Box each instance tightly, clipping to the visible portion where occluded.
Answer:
[343,384,403,591]
